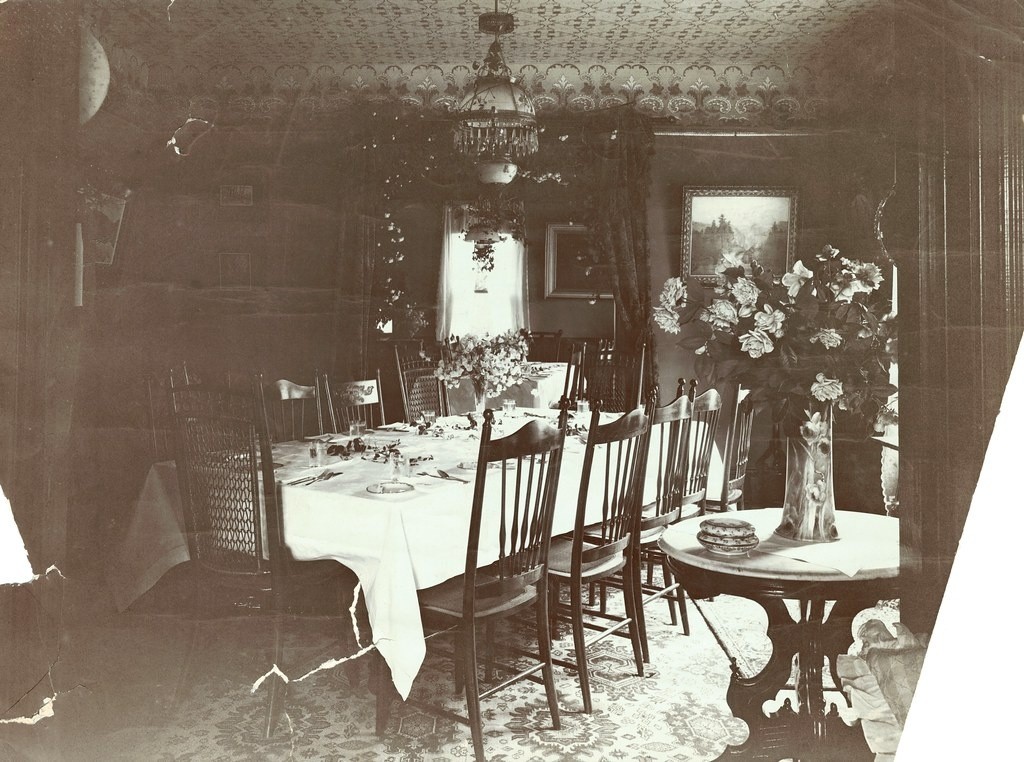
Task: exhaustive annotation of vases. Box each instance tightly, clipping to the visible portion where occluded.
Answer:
[767,402,842,542]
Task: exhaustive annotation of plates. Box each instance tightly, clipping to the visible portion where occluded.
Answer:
[366,480,415,494]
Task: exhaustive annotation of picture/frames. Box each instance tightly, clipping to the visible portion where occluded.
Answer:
[541,222,617,300]
[220,185,253,206]
[218,251,252,291]
[681,184,802,286]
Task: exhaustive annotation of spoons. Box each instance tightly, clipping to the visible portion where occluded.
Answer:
[318,472,333,482]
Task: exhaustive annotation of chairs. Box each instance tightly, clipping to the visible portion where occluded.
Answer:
[170,326,754,760]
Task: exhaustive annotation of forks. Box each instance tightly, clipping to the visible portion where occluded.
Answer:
[306,469,331,486]
[437,469,470,484]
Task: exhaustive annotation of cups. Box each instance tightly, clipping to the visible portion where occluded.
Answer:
[503,399,516,414]
[310,440,318,467]
[316,443,327,467]
[420,409,437,427]
[349,421,358,438]
[357,420,366,439]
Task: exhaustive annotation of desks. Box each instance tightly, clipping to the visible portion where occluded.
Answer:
[106,405,726,702]
[655,502,900,762]
[438,361,590,412]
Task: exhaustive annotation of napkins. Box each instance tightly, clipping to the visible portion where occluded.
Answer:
[454,1,539,243]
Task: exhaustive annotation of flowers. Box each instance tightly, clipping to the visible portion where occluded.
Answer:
[651,247,899,451]
